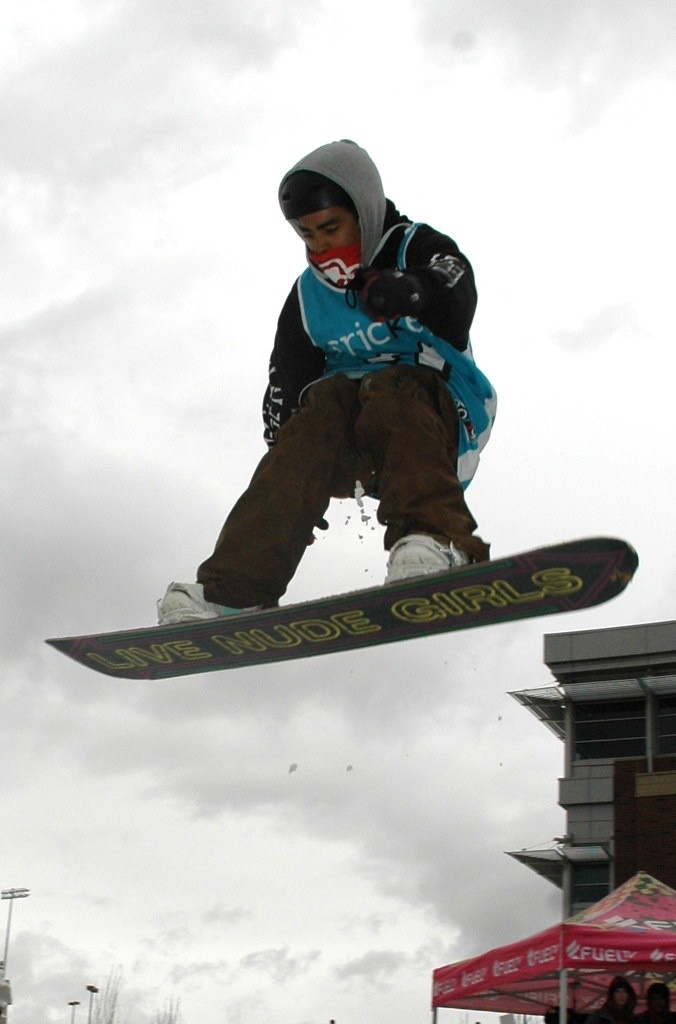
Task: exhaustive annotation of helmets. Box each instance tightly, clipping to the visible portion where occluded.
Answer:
[280,171,349,221]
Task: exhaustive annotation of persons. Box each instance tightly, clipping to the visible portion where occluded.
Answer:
[159,136,497,621]
[545,975,676,1024]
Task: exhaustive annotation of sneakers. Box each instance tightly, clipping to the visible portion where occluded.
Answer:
[385,531,470,581]
[161,581,262,619]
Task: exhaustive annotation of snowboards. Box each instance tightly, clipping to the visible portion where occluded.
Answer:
[43,536,639,684]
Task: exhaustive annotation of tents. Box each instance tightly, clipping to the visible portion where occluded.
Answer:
[430,870,676,1024]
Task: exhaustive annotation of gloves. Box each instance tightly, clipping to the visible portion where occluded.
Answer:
[359,269,422,322]
